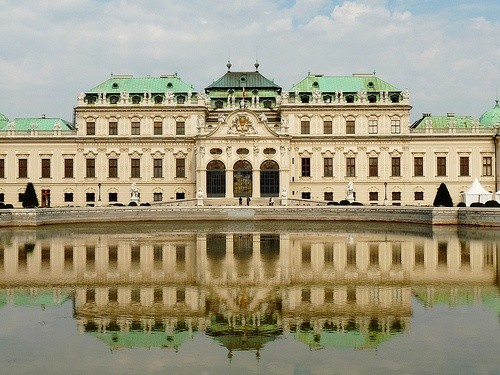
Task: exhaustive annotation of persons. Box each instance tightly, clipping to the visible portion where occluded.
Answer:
[246,197,251,206]
[268,196,274,206]
[238,195,242,206]
